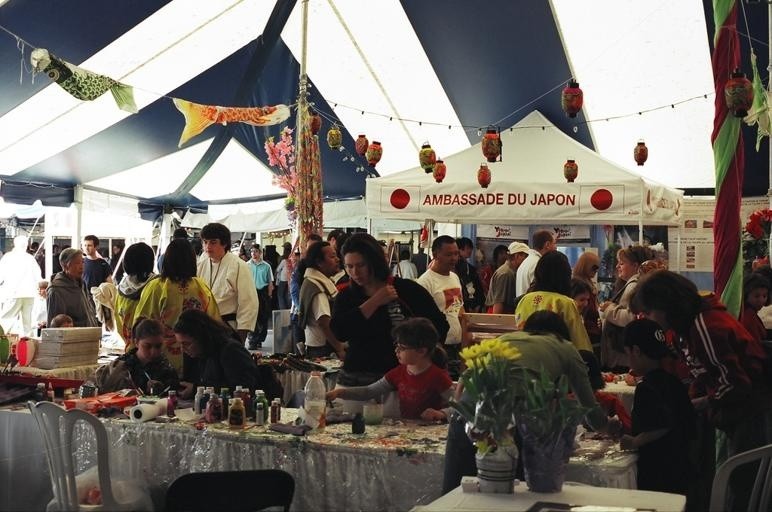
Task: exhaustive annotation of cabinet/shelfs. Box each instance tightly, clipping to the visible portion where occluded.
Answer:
[35,382,55,402]
[352,412,365,434]
[304,370,326,433]
[168,386,281,428]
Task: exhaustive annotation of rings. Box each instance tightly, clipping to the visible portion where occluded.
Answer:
[158,385,171,397]
[136,387,146,398]
[150,387,154,396]
[144,371,152,383]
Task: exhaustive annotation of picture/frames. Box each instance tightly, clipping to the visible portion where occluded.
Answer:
[602,371,638,419]
[413,471,690,512]
[0,389,647,511]
[0,363,103,392]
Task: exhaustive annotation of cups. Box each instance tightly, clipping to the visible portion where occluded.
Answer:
[394,341,415,352]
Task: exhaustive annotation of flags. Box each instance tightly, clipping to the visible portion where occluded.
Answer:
[474,441,519,494]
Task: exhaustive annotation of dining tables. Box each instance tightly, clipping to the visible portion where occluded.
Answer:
[516,362,593,493]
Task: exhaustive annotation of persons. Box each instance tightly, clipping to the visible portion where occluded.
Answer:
[0,222,772,496]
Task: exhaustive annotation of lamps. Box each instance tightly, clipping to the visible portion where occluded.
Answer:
[0,410,55,512]
[25,401,156,512]
[162,469,294,512]
[708,445,772,512]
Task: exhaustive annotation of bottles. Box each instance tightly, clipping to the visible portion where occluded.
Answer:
[625,318,667,349]
[507,242,529,255]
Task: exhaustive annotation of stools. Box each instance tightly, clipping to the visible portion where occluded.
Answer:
[443,342,460,349]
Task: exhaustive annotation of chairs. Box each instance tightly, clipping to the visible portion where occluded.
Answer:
[247,342,263,350]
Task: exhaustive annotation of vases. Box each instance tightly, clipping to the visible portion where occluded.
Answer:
[742,207,772,276]
[448,336,526,455]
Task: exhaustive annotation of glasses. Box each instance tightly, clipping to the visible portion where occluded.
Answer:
[573,124,579,134]
